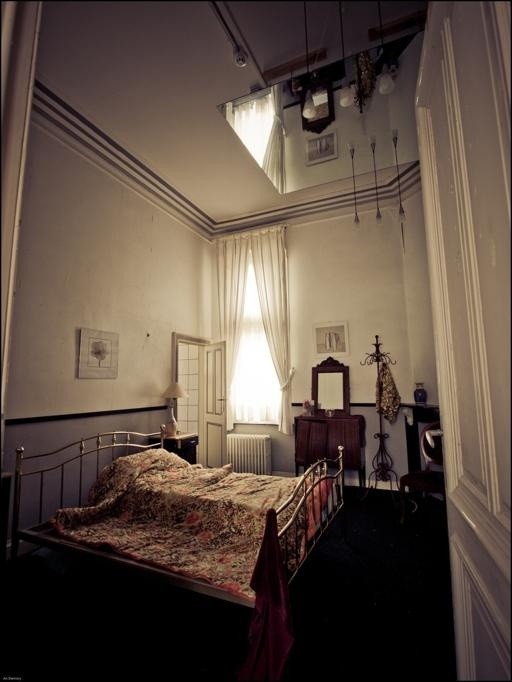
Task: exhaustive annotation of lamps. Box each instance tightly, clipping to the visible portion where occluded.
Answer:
[161,379,189,436]
[348,176,408,229]
[299,1,396,122]
[346,128,400,175]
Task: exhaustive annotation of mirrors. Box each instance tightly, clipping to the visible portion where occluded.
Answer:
[298,81,338,134]
[213,25,424,195]
[311,355,350,417]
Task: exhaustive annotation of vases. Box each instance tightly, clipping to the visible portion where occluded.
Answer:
[413,381,428,407]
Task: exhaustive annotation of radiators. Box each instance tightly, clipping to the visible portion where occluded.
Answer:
[225,433,273,476]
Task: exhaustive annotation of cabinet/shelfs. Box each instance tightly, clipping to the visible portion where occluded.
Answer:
[294,420,365,493]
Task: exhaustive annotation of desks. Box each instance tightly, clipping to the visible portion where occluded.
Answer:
[399,401,446,506]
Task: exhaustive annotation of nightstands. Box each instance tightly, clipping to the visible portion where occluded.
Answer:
[146,431,199,464]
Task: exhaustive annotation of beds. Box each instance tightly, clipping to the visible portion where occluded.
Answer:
[9,426,347,682]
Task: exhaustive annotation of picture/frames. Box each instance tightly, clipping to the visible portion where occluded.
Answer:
[312,319,350,357]
[77,326,121,380]
[302,129,340,168]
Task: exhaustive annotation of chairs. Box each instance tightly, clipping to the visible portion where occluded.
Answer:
[399,421,445,524]
